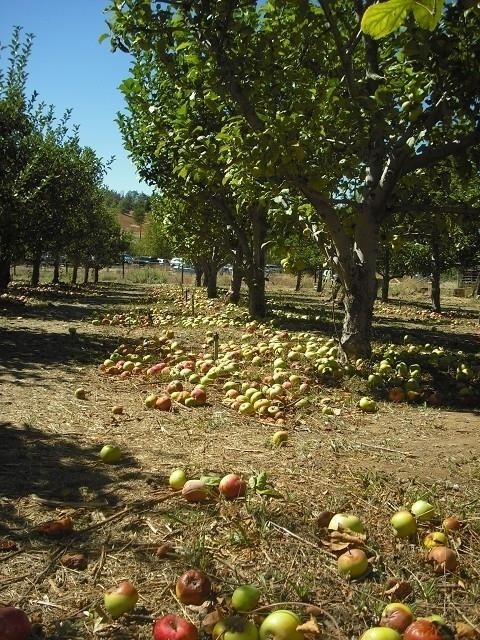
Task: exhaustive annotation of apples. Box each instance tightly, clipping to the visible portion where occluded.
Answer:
[0,447,480,638]
[0,280,480,447]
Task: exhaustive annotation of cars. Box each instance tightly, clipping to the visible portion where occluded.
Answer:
[115,252,281,278]
